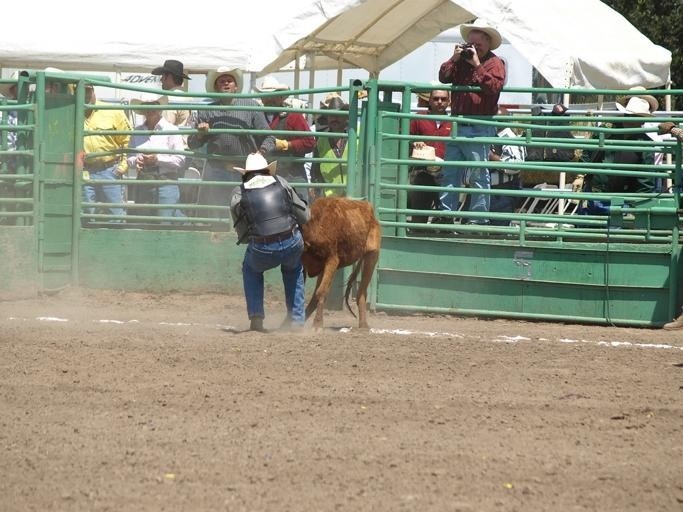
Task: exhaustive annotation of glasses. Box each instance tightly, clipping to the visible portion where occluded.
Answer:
[433,96,447,103]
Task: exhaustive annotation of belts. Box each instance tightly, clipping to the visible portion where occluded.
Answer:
[254,231,291,245]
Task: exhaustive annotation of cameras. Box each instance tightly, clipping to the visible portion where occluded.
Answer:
[460,45,473,60]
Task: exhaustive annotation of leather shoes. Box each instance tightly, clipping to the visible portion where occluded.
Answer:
[250,317,264,331]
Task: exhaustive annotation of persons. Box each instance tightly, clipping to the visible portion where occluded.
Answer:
[433,23,511,224]
[231,152,311,332]
[0,59,360,223]
[492,86,683,213]
[410,89,454,235]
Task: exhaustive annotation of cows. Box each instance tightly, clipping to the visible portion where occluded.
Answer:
[297,195,382,330]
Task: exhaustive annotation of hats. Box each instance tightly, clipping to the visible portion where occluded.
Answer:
[618,85,659,111]
[414,78,451,104]
[0,70,36,98]
[232,151,277,176]
[45,67,74,95]
[408,145,444,163]
[614,97,656,118]
[459,18,502,50]
[129,59,348,126]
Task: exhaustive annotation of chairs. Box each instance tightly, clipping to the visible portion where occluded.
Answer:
[132,166,202,217]
[507,180,580,237]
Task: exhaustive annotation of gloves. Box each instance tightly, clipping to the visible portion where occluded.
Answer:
[656,121,675,135]
[275,139,288,153]
[571,174,583,192]
